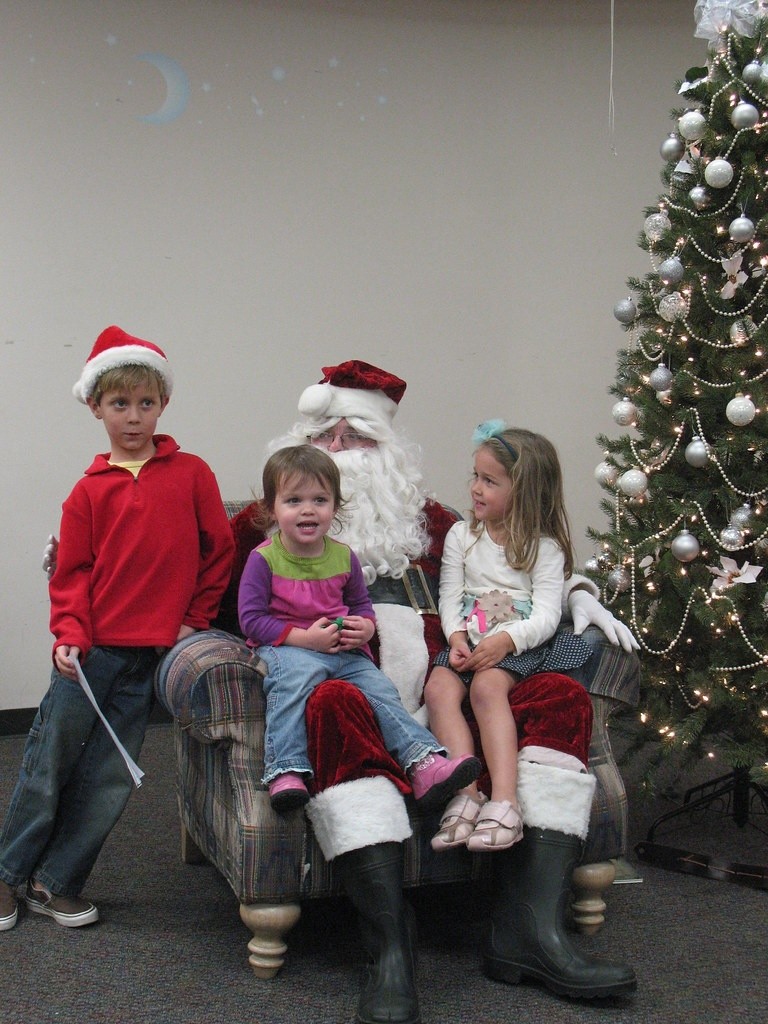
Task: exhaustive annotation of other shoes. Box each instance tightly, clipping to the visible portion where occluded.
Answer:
[269,772,311,809]
[408,753,483,805]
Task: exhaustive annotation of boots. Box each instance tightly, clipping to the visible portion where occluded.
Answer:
[478,761,639,1001]
[307,776,420,1024]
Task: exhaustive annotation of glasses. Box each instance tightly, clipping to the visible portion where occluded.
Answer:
[305,432,373,451]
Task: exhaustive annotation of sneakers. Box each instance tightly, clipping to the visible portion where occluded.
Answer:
[0,878,18,930]
[467,799,525,850]
[430,791,489,849]
[26,880,98,927]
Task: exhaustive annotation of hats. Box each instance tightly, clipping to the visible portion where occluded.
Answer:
[73,325,173,403]
[297,360,408,425]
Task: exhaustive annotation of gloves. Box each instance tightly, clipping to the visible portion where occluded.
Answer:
[41,534,56,580]
[569,590,640,654]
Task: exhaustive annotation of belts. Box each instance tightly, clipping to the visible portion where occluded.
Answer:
[363,563,443,615]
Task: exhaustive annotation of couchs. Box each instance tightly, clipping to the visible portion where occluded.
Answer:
[149,499,642,979]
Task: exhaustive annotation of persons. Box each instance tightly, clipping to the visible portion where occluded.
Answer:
[1,323,237,929]
[426,420,573,851]
[237,446,482,817]
[44,359,641,1024]
